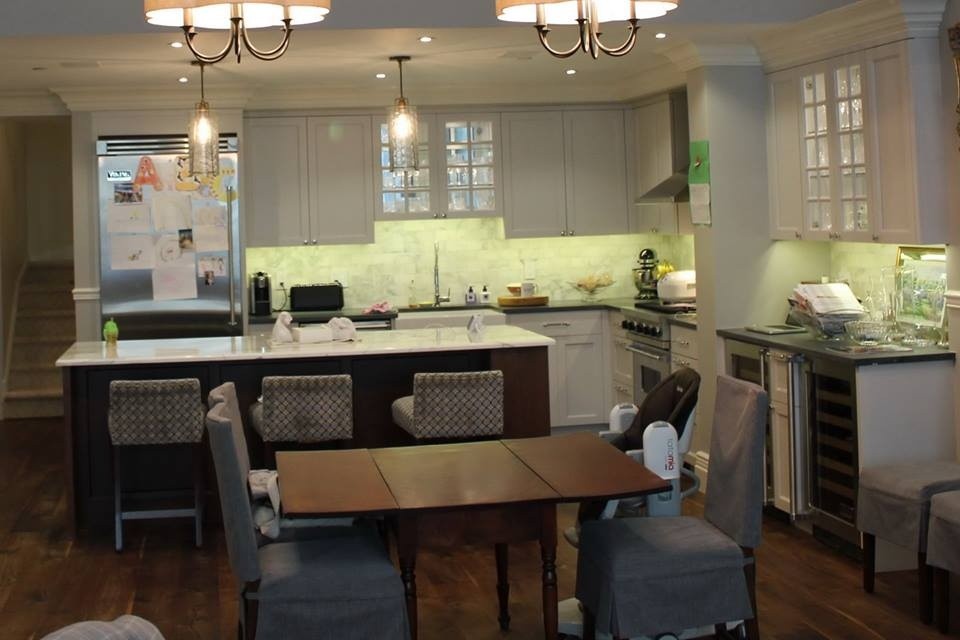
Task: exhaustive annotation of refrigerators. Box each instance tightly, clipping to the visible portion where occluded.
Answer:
[96,138,244,341]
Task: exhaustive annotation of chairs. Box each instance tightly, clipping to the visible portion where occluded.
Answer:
[204,366,772,640]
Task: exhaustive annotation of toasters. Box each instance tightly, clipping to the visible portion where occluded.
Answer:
[290,280,344,311]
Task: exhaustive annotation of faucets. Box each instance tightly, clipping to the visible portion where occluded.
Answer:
[433,241,450,308]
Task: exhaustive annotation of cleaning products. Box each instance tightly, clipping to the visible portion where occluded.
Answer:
[407,279,417,308]
[465,285,478,304]
[479,284,491,304]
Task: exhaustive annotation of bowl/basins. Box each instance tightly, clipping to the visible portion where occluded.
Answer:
[506,286,521,297]
[890,290,948,346]
[845,320,893,346]
[818,317,862,340]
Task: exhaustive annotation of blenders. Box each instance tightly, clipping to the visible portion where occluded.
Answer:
[631,249,658,299]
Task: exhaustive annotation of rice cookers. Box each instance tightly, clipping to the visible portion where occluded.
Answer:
[657,270,696,302]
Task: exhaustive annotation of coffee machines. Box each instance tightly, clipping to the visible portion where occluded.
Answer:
[250,271,272,316]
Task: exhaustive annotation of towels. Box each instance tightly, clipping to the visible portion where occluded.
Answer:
[329,317,361,342]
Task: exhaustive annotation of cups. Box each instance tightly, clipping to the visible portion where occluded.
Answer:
[521,282,536,297]
[880,265,905,337]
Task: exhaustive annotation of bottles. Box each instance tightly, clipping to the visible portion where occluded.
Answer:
[103,321,118,345]
[408,279,417,307]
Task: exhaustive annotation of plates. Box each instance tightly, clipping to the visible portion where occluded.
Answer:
[419,303,433,307]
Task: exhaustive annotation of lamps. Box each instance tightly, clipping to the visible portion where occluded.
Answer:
[388,52,422,173]
[494,0,681,59]
[895,246,946,266]
[188,58,219,175]
[142,1,332,64]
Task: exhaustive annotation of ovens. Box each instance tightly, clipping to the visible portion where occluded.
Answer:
[625,342,671,408]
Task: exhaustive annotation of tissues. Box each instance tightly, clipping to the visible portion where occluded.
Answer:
[291,325,332,344]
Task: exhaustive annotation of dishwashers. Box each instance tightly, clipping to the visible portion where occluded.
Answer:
[300,320,390,331]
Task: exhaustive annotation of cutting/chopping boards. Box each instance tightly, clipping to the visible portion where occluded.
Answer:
[498,296,549,306]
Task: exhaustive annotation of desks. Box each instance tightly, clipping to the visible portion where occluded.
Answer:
[275,428,676,640]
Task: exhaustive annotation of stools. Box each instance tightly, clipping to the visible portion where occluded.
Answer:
[104,377,208,547]
[392,371,507,441]
[253,376,356,473]
[924,489,960,632]
[854,458,960,623]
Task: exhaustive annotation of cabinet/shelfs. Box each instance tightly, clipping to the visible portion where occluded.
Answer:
[634,101,677,236]
[763,67,804,240]
[512,311,606,427]
[372,114,503,221]
[768,346,801,517]
[671,325,701,455]
[863,39,921,244]
[806,50,875,245]
[504,110,626,237]
[609,310,633,403]
[244,117,375,245]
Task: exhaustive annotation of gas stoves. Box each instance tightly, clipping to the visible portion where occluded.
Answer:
[620,301,696,348]
